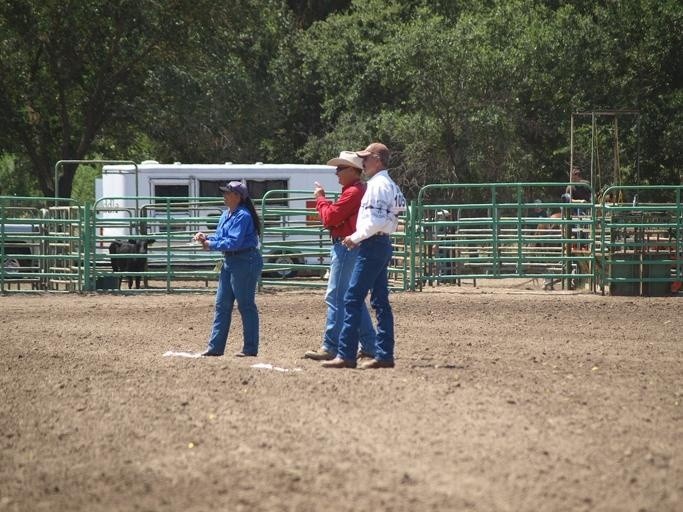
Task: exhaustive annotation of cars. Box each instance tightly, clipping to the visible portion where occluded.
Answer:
[0,224,45,279]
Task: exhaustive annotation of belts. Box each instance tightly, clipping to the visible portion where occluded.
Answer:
[375,232,390,237]
[331,236,346,244]
[224,247,256,256]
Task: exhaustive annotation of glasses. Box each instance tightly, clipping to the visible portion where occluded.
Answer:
[336,166,350,171]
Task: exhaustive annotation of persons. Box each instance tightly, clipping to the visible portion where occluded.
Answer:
[562,167,589,251]
[320,144,407,369]
[191,181,264,358]
[528,199,545,231]
[423,210,450,284]
[304,149,375,361]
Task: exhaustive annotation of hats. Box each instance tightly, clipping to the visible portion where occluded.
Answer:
[326,150,365,170]
[218,181,249,200]
[355,142,389,159]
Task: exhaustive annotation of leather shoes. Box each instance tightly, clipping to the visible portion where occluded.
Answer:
[355,350,375,359]
[305,347,337,360]
[320,355,357,368]
[362,359,394,369]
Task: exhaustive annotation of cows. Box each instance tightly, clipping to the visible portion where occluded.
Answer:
[109,238,156,289]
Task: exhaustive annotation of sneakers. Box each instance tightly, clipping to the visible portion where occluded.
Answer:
[234,351,257,357]
[201,350,224,355]
